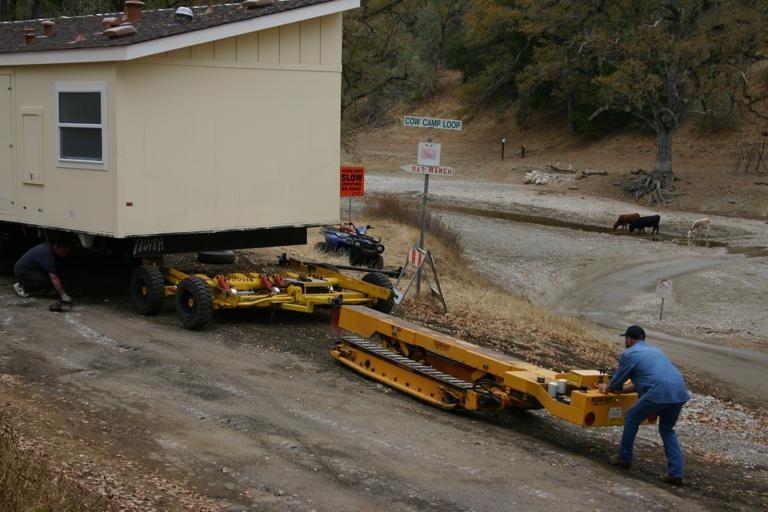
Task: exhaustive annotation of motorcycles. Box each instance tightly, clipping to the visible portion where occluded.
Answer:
[314,221,387,269]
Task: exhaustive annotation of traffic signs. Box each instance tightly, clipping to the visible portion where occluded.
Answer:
[400,164,453,177]
[403,115,462,132]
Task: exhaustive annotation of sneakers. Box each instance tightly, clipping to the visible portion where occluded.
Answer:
[13,282,29,298]
[607,454,631,468]
[657,472,682,487]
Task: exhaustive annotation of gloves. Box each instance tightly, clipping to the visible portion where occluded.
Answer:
[61,293,72,302]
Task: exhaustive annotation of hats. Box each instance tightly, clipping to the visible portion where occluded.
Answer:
[619,325,645,340]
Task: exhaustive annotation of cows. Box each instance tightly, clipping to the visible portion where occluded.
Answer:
[614,214,640,231]
[629,215,660,236]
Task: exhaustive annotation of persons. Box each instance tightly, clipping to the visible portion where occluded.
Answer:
[598,325,689,486]
[13,240,74,302]
